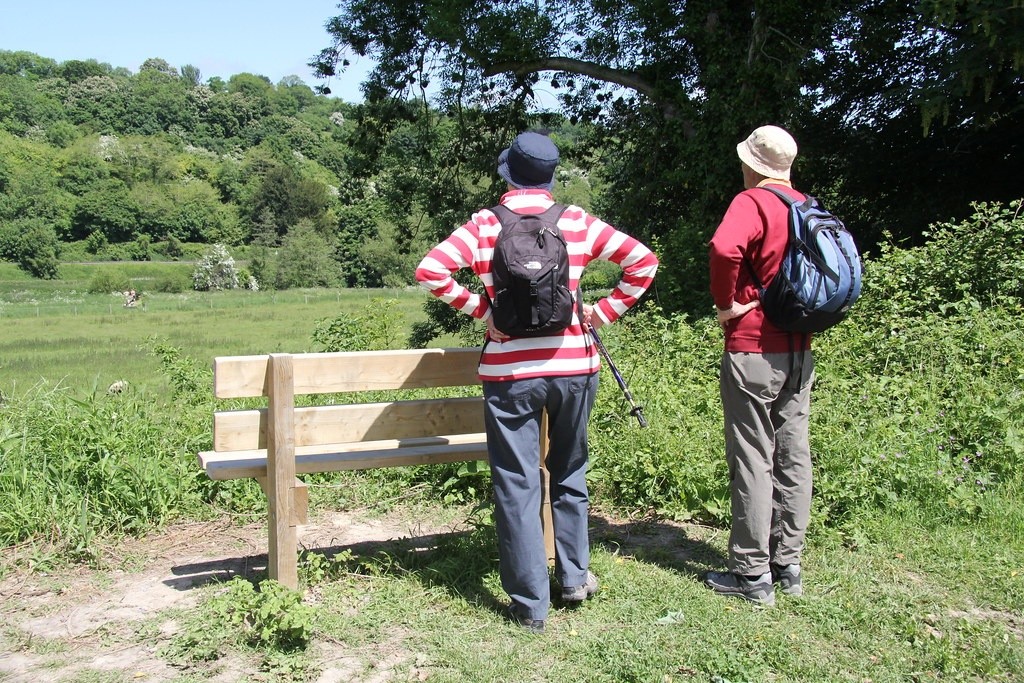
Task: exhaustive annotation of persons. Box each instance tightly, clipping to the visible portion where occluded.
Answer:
[701,125,819,607]
[414,133,659,629]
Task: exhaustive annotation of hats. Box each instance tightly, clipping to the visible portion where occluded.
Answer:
[736,125,798,181]
[496,132,559,191]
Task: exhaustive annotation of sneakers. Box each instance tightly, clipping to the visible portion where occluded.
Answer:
[702,569,774,610]
[561,569,597,609]
[768,562,803,597]
[508,599,543,631]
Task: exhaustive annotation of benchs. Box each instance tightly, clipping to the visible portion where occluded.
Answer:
[197,347,565,591]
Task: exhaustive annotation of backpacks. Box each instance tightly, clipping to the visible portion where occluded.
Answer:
[742,187,862,333]
[482,204,575,336]
